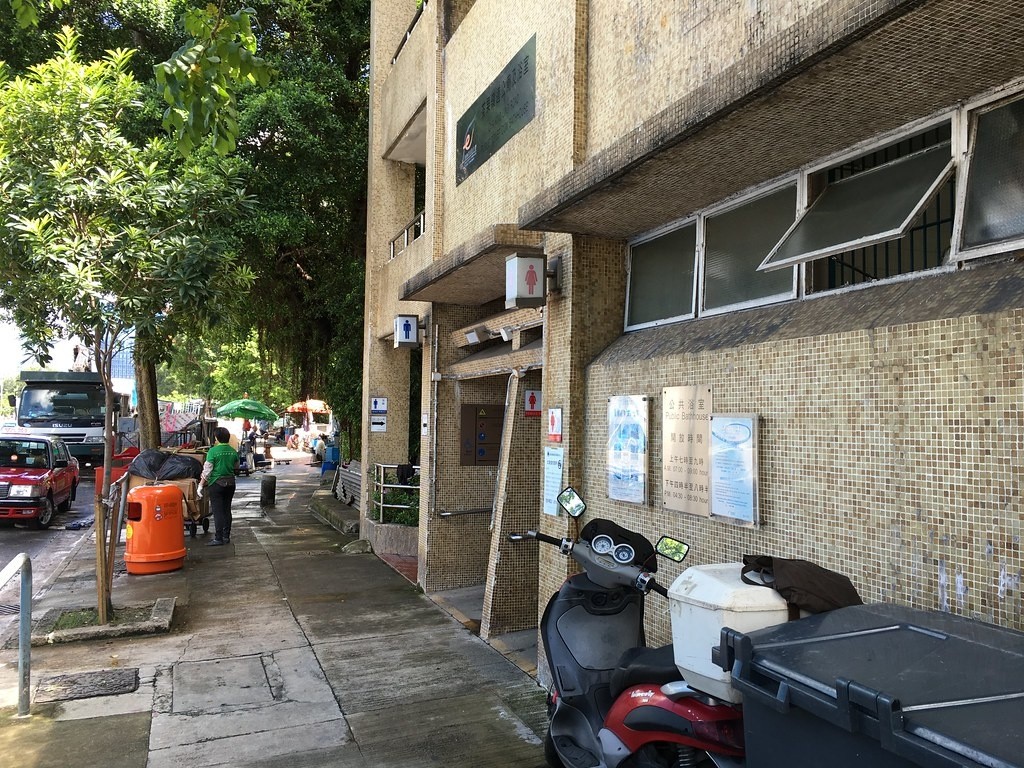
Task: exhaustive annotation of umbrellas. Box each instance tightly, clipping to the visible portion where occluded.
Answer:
[217,399,279,438]
[287,399,330,414]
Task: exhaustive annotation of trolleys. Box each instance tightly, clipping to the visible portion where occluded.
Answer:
[183,485,214,537]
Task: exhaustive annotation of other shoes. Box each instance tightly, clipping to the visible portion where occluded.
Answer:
[222,538,229,543]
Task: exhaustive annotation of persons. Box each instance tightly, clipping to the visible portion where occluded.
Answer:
[197,428,240,546]
[248,420,331,463]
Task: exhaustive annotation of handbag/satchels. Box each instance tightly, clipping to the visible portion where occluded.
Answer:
[740,553,864,618]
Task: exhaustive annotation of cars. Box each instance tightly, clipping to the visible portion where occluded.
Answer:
[0,436,80,530]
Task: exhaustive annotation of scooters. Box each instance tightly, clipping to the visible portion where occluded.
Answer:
[505,484,814,768]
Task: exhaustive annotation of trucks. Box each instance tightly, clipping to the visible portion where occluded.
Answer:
[8,369,131,464]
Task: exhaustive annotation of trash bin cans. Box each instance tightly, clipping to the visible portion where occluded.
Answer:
[123,484,187,575]
[711,601,1024,768]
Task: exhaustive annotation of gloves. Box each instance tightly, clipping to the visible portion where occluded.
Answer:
[196,484,205,499]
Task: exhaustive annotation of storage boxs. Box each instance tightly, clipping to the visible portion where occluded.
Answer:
[129,449,209,520]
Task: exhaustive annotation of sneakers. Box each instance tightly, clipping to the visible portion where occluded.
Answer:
[205,541,223,546]
